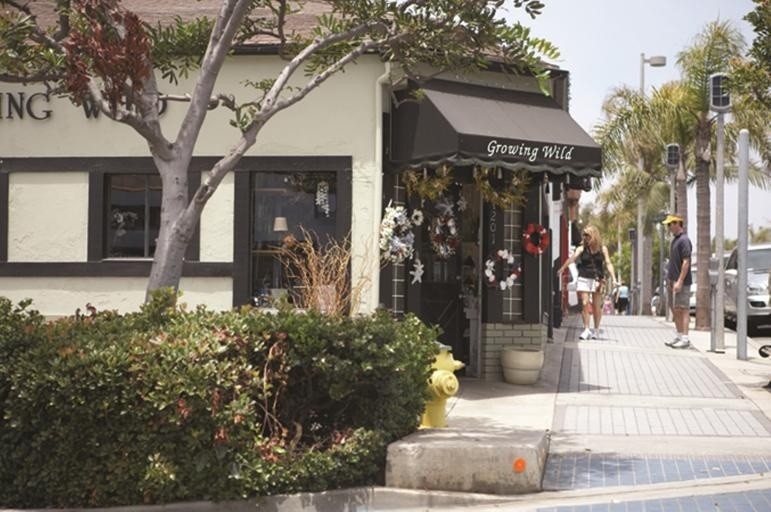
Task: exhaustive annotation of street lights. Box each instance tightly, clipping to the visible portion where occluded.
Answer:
[636,51,666,310]
[707,71,733,355]
[665,142,680,217]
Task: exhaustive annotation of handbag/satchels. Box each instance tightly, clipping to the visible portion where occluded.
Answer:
[576,276,596,293]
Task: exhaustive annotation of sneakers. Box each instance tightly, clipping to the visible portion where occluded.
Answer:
[591,330,599,339]
[664,336,681,346]
[672,339,690,348]
[579,328,591,339]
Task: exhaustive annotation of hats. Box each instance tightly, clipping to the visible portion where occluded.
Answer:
[661,215,683,226]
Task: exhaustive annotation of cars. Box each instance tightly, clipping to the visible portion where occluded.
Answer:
[688,243,770,337]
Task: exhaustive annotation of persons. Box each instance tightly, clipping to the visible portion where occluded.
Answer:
[602,295,614,313]
[659,212,695,349]
[615,282,632,316]
[610,283,620,309]
[556,223,622,340]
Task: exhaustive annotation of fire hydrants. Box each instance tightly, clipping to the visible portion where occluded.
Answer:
[418,339,465,429]
